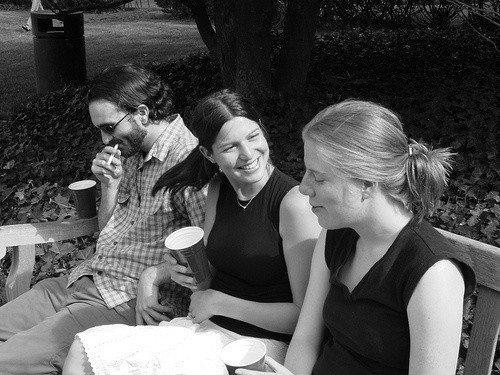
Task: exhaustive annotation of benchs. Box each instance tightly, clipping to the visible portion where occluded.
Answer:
[0,216,500,375]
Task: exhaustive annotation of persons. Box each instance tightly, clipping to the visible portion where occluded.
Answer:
[235,97,477,375]
[62,87,324,375]
[0,62,223,375]
[21,0,44,32]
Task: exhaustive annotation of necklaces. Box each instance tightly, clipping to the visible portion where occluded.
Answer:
[135,151,143,207]
[237,177,269,209]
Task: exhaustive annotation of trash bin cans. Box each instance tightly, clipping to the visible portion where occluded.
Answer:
[30,9,87,93]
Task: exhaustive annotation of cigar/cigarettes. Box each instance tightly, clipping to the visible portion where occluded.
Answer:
[106,144,119,166]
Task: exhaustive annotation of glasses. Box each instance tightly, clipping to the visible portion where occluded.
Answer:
[90,109,137,136]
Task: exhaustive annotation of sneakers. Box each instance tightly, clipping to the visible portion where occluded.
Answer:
[22,24,31,31]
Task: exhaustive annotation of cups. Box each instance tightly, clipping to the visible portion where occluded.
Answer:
[165,226,211,285]
[221,339,268,375]
[68,180,97,219]
